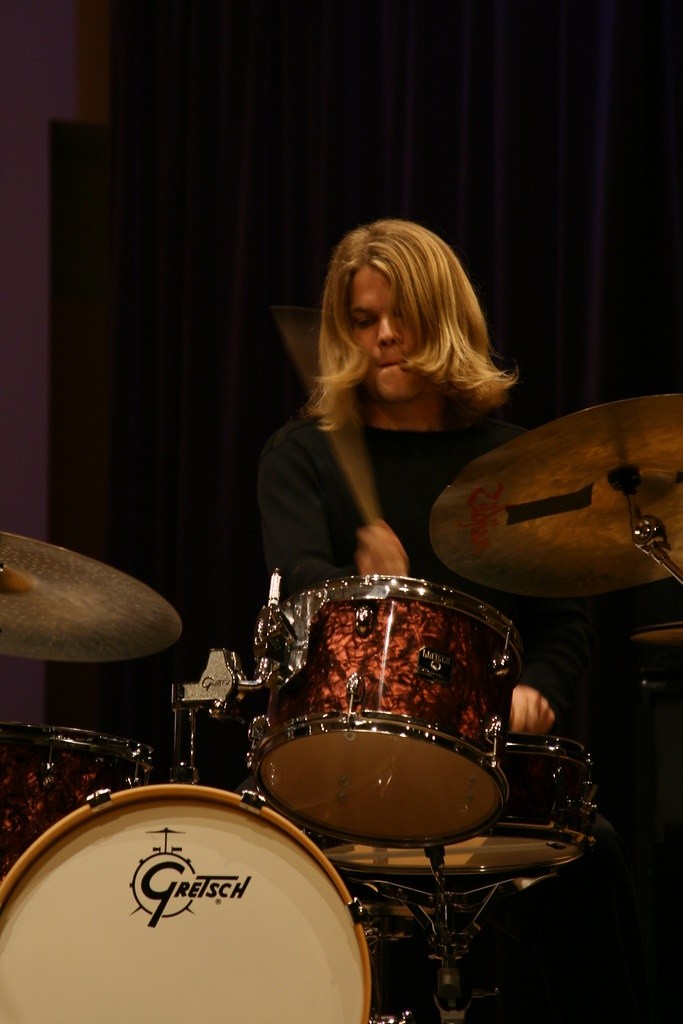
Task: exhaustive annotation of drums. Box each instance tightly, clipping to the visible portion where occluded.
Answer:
[2,783,375,1023]
[264,568,520,841]
[0,723,152,886]
[300,730,591,878]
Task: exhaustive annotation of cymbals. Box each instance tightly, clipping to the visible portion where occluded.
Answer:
[1,527,187,665]
[630,618,682,644]
[429,393,682,601]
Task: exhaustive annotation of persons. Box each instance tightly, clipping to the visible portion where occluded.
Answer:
[256,220,556,738]
